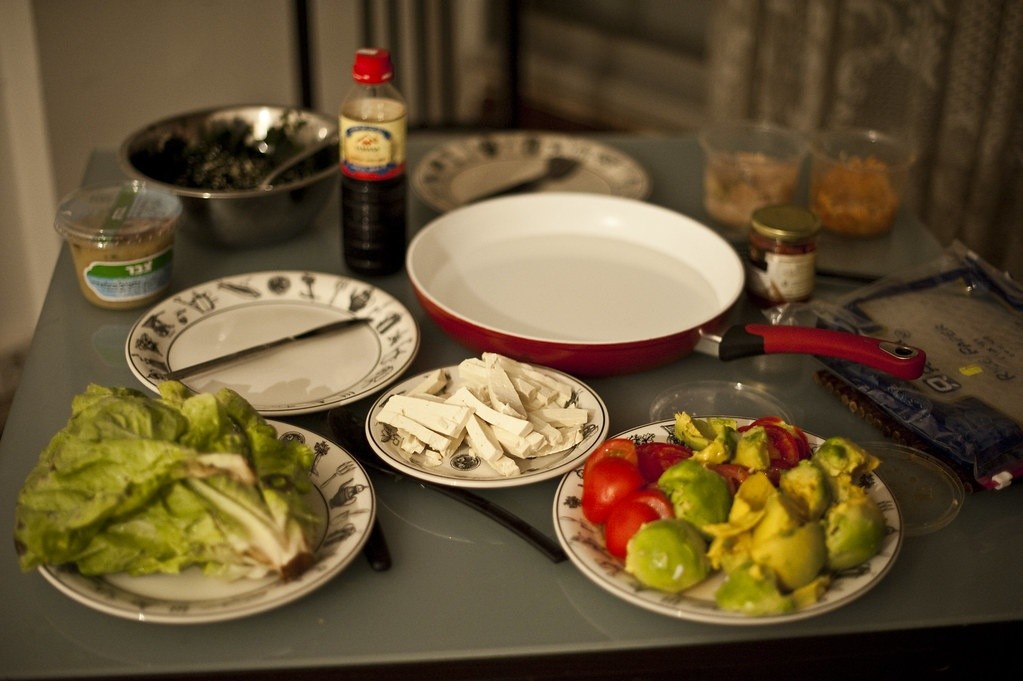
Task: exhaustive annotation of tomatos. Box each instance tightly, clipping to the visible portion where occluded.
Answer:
[583,416,811,557]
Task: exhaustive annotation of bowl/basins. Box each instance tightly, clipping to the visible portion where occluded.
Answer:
[694,120,809,222]
[53,182,175,311]
[806,129,919,240]
[117,103,339,246]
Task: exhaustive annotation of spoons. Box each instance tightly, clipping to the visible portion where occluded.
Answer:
[327,404,568,565]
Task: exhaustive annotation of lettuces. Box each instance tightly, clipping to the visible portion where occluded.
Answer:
[14,380,315,571]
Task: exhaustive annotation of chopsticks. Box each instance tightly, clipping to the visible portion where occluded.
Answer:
[812,370,968,489]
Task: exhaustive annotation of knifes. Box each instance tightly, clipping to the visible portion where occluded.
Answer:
[165,318,372,382]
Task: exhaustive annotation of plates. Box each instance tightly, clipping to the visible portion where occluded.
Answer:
[366,365,613,489]
[552,417,903,625]
[38,421,375,623]
[410,130,649,212]
[125,270,421,416]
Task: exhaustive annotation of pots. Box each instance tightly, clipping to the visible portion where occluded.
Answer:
[406,188,926,380]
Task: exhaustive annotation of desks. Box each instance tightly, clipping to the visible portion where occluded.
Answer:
[0,134,1023,681]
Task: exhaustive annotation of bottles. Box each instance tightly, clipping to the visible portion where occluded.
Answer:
[338,49,405,275]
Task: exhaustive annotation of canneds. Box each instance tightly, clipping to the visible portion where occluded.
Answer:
[746,204,821,301]
